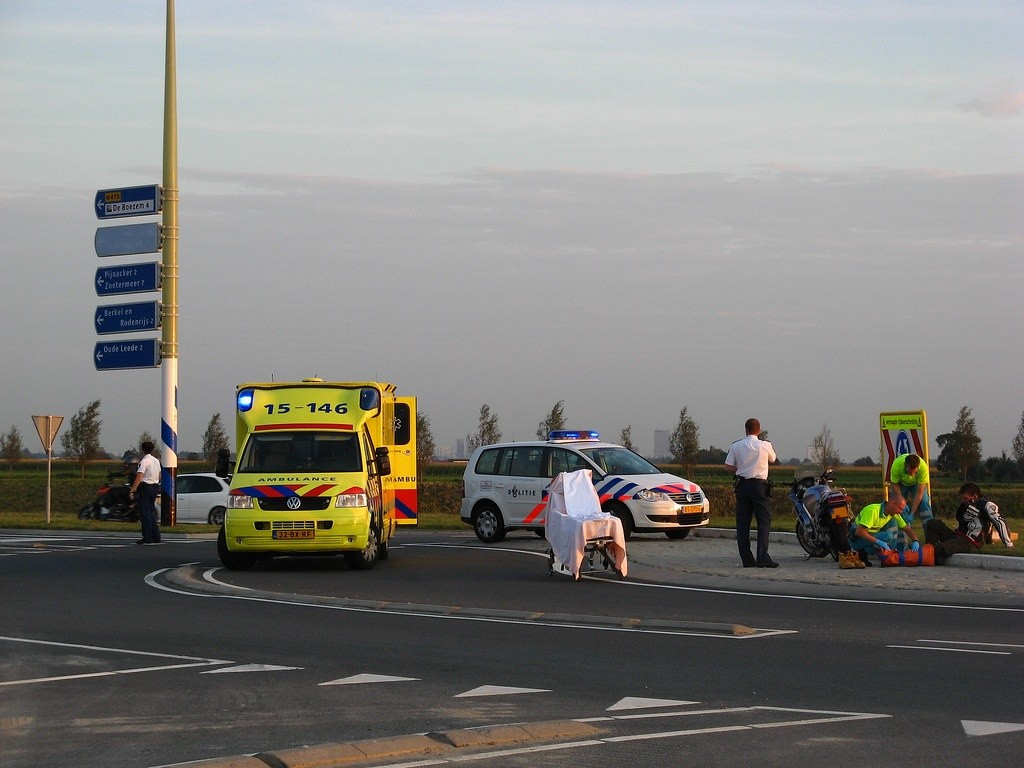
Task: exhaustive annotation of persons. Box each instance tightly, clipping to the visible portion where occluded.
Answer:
[725,418,780,569]
[879,482,1016,568]
[848,495,920,567]
[890,453,933,533]
[129,442,162,545]
[96,483,109,496]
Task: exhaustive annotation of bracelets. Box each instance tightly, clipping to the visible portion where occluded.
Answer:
[130,490,134,494]
[912,540,919,543]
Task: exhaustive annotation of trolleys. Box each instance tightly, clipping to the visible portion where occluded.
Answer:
[545,470,627,580]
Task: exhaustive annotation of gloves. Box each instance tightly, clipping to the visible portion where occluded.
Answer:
[875,540,893,551]
[911,541,919,552]
[902,505,910,514]
[905,513,913,523]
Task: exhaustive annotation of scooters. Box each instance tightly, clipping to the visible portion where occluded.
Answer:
[79,466,143,524]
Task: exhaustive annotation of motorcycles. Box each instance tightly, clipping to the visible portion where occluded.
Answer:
[790,462,856,563]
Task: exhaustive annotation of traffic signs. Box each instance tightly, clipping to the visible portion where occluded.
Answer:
[94,183,162,221]
[94,338,161,373]
[93,300,163,336]
[94,261,162,298]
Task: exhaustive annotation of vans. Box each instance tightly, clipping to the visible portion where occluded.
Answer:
[458,430,710,544]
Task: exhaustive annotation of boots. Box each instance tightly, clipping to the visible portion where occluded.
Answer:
[838,551,855,568]
[847,551,866,568]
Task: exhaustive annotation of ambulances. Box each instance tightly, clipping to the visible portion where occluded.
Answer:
[214,376,420,568]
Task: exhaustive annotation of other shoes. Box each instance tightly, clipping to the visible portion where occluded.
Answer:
[137,536,153,544]
[743,558,756,567]
[936,556,943,565]
[859,552,872,567]
[756,560,779,568]
[152,537,161,543]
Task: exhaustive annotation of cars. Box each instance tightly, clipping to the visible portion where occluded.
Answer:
[100,473,233,527]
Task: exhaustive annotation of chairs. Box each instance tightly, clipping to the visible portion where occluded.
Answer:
[551,457,563,477]
[504,457,517,475]
[548,472,627,576]
[573,457,587,471]
[264,442,290,469]
